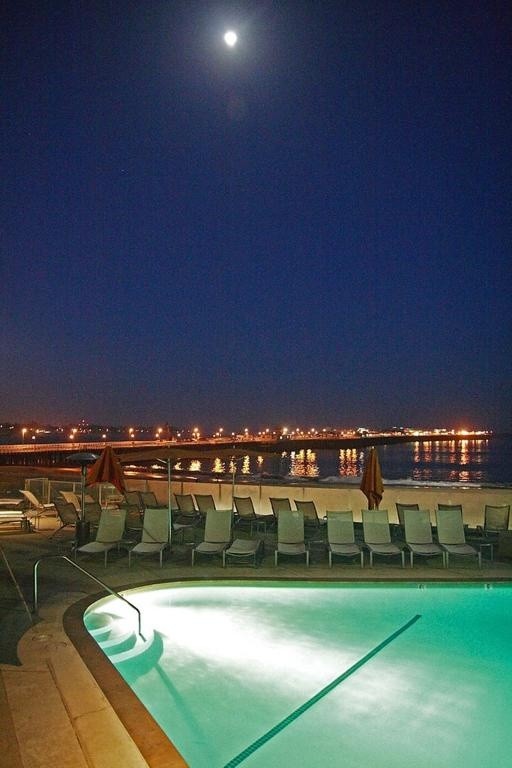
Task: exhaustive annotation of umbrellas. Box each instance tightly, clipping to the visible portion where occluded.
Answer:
[359,448,385,509]
[120,444,212,547]
[85,444,128,503]
[200,445,281,511]
